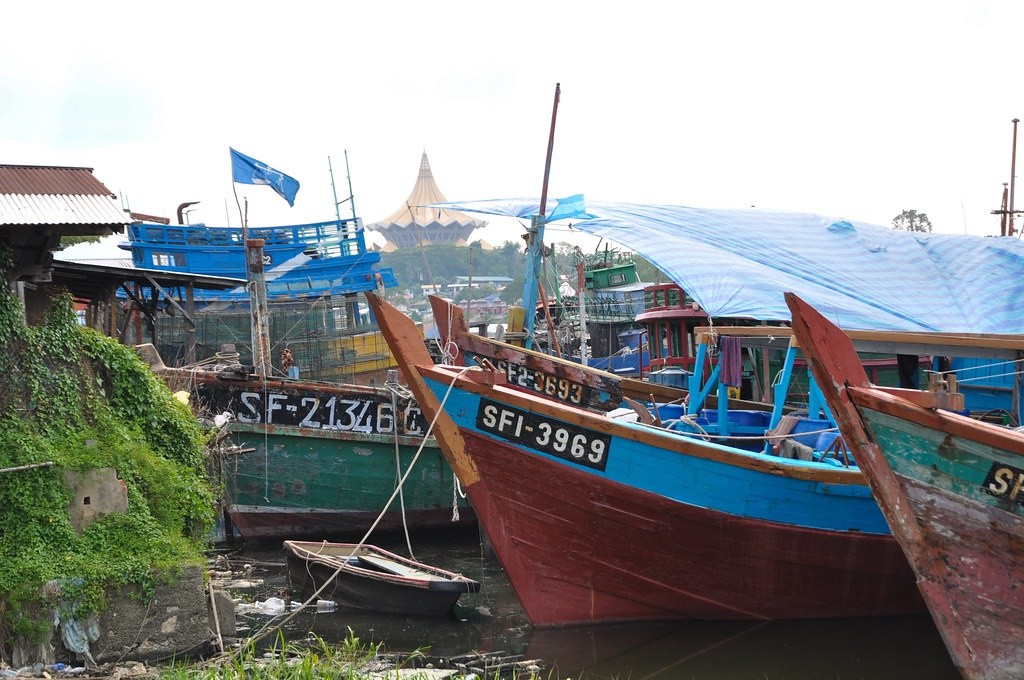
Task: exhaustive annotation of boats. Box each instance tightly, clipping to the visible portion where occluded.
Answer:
[65,83,929,628]
[783,290,1023,680]
